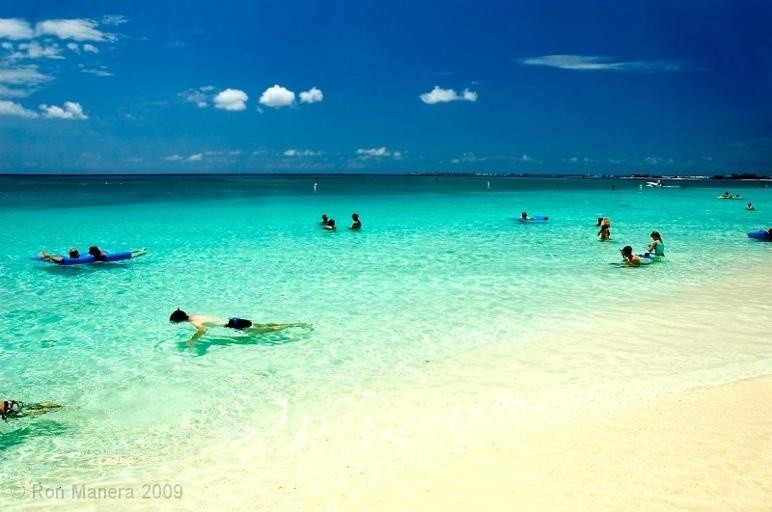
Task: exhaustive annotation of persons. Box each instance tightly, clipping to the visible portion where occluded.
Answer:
[171,307,314,345]
[0,400,67,423]
[520,179,772,267]
[40,245,146,265]
[317,213,361,233]
